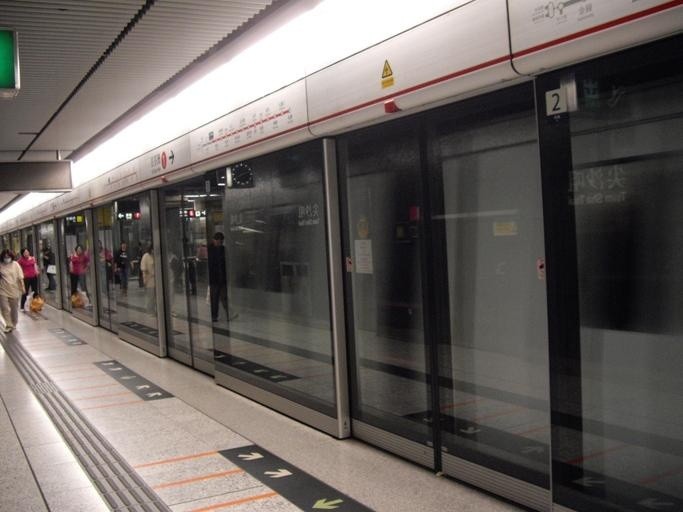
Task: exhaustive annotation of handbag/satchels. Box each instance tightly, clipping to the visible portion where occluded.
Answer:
[46,265,56,275]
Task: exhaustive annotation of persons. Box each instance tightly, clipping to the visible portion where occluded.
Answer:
[41,232,241,324]
[0,247,45,335]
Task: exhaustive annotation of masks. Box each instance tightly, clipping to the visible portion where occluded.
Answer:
[4,257,12,264]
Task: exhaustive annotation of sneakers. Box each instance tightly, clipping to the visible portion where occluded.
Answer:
[227,313,239,321]
[3,325,16,333]
[171,311,179,317]
